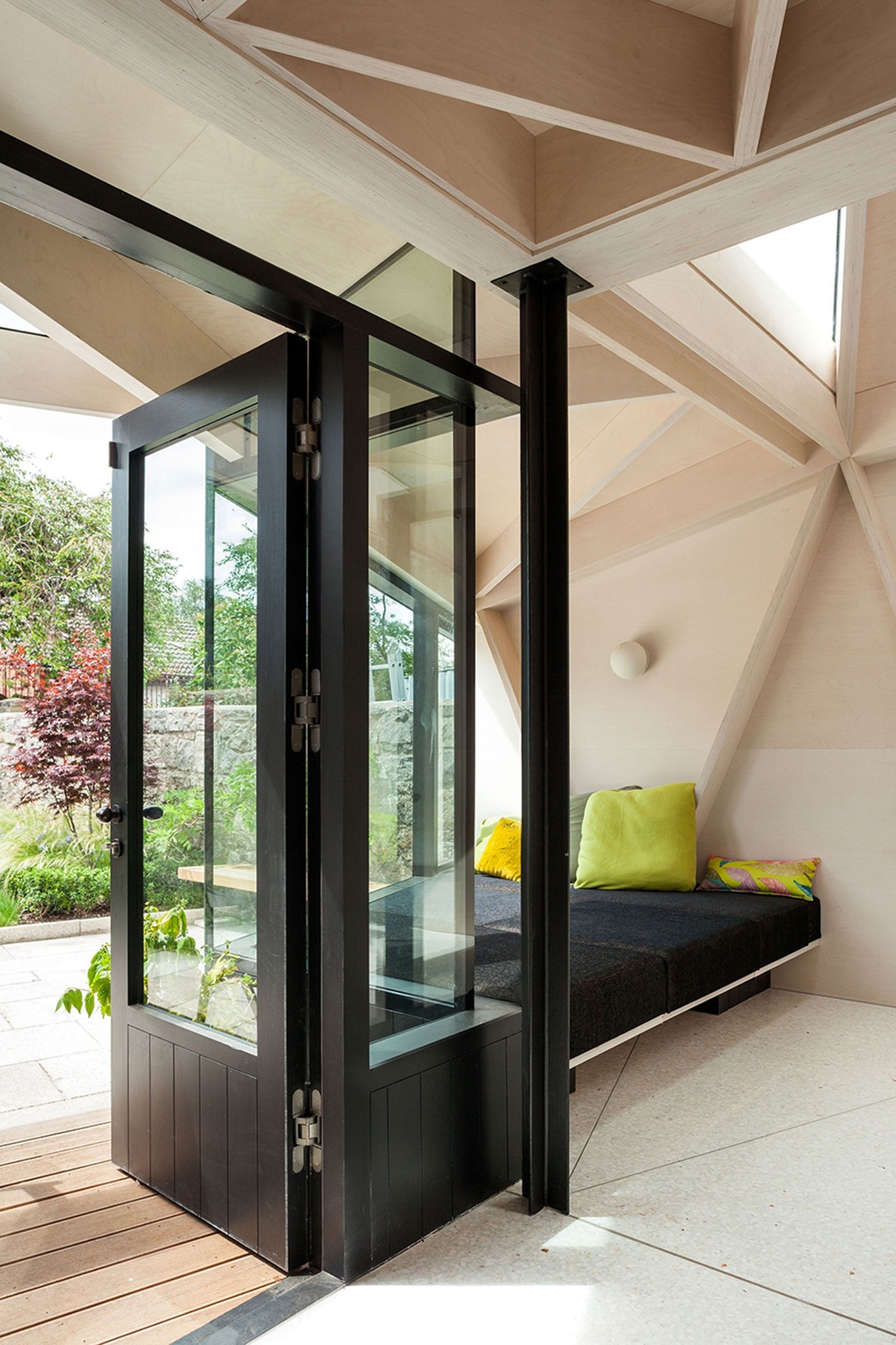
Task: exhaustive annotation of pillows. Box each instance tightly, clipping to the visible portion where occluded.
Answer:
[696,853,821,901]
[475,816,521,881]
[573,782,698,891]
[568,786,643,884]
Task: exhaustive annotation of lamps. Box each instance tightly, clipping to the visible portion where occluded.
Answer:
[610,640,650,679]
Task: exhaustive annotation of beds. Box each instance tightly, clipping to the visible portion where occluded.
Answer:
[370,852,823,1096]
